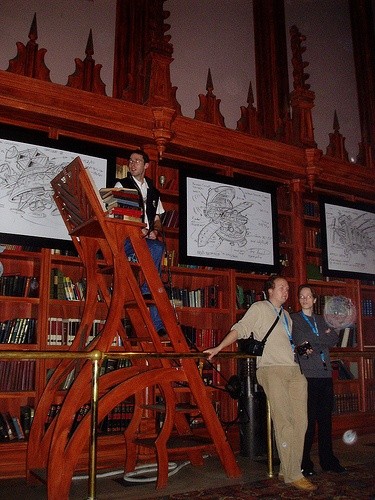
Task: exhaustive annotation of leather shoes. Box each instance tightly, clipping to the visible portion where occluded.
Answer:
[323,464,347,474]
[302,468,317,476]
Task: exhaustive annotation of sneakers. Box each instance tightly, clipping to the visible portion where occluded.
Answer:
[285,475,317,491]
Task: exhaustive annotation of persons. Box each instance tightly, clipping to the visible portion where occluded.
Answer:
[203,275,317,490]
[289,285,345,477]
[112,149,167,338]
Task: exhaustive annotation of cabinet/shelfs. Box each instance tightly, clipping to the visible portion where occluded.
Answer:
[0,247,375,480]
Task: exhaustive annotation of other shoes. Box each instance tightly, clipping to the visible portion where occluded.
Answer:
[158,329,168,337]
[142,294,152,300]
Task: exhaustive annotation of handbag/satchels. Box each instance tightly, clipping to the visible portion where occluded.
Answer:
[238,337,264,356]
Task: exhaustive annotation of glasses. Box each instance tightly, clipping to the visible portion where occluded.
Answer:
[129,158,145,164]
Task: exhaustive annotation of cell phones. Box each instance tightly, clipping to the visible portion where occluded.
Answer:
[297,342,312,355]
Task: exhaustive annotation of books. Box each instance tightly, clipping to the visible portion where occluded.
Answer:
[0,188,375,442]
